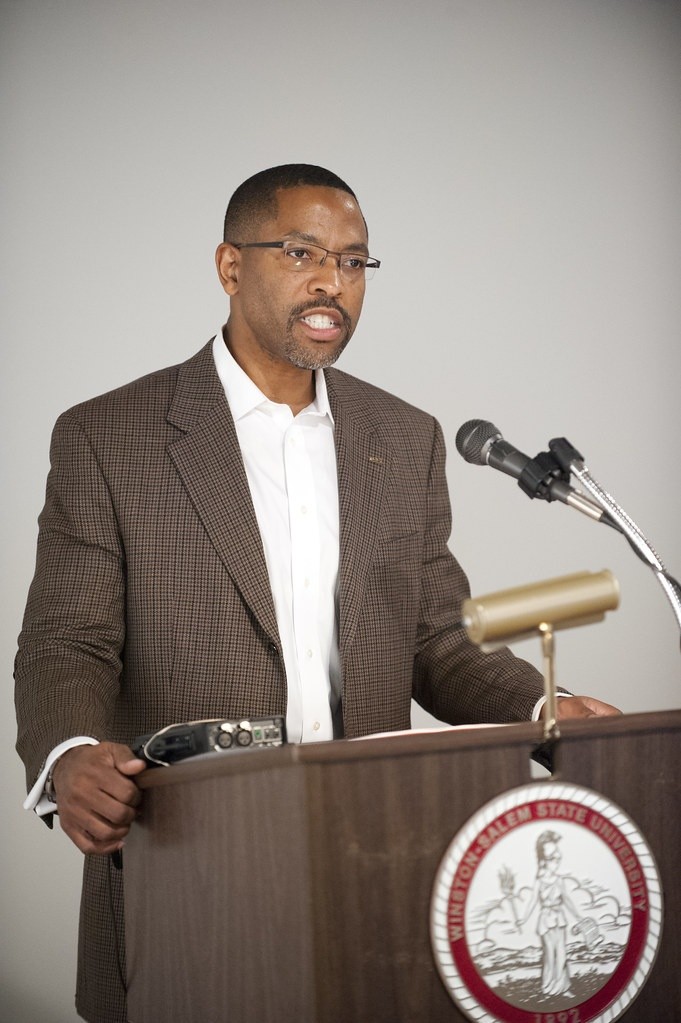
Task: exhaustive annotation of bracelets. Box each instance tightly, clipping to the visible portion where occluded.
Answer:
[45,757,57,804]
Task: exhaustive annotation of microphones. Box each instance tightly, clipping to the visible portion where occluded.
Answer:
[455,418,623,534]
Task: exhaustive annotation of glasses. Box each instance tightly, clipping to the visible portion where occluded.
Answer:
[233,241,381,280]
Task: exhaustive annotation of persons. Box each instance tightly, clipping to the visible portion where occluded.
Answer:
[12,164,622,1023]
